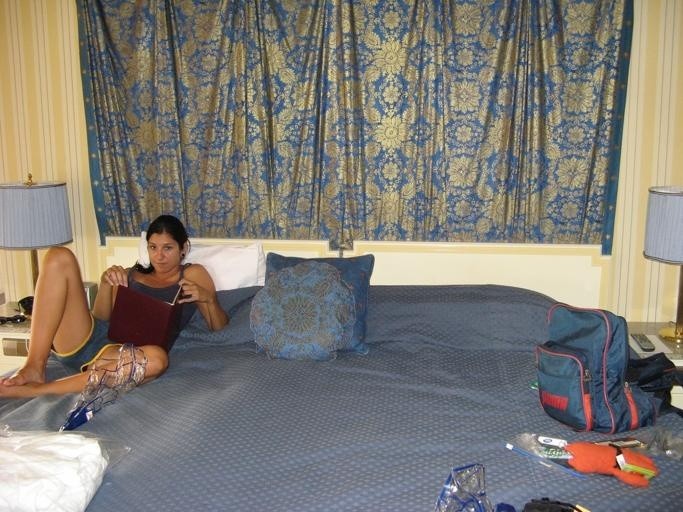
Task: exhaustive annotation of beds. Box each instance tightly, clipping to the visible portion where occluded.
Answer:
[1,235,574,490]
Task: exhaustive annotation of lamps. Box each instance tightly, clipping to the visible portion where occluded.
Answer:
[1,171,75,320]
[646,187,682,345]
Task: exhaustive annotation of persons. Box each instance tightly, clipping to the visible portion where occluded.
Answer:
[0,215,228,399]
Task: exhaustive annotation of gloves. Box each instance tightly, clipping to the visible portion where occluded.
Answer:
[542,438,657,488]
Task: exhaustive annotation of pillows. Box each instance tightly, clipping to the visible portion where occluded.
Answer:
[138,229,376,364]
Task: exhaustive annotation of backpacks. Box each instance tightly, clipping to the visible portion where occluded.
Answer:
[534,302,683,434]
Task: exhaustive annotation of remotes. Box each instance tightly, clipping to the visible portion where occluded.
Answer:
[631,333,655,352]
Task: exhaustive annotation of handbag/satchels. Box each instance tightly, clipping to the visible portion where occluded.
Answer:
[108,271,183,355]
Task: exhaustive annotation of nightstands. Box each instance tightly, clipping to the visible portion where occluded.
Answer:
[1,325,54,376]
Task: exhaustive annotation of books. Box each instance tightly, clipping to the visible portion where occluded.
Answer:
[107,284,184,347]
